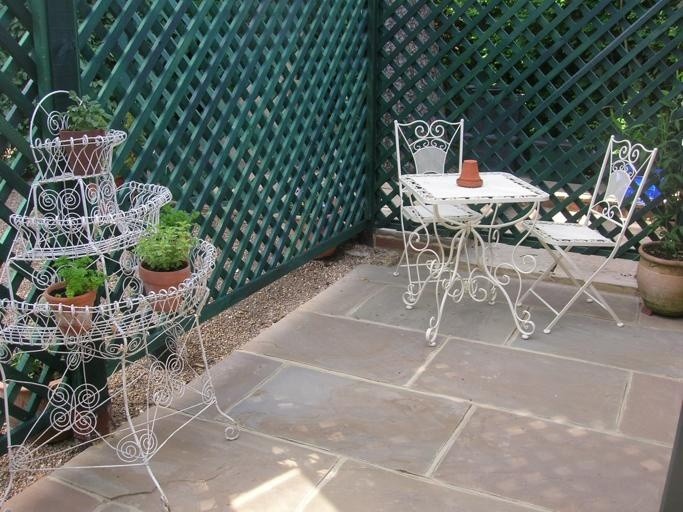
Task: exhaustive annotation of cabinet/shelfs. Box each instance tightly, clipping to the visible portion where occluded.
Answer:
[1,79,244,511]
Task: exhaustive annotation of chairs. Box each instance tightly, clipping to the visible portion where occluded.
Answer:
[392,114,484,311]
[518,134,659,331]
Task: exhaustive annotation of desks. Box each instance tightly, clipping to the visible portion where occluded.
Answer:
[399,172,553,348]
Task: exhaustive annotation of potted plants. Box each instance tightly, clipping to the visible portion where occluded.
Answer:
[59,88,113,176]
[39,200,203,337]
[611,95,682,320]
